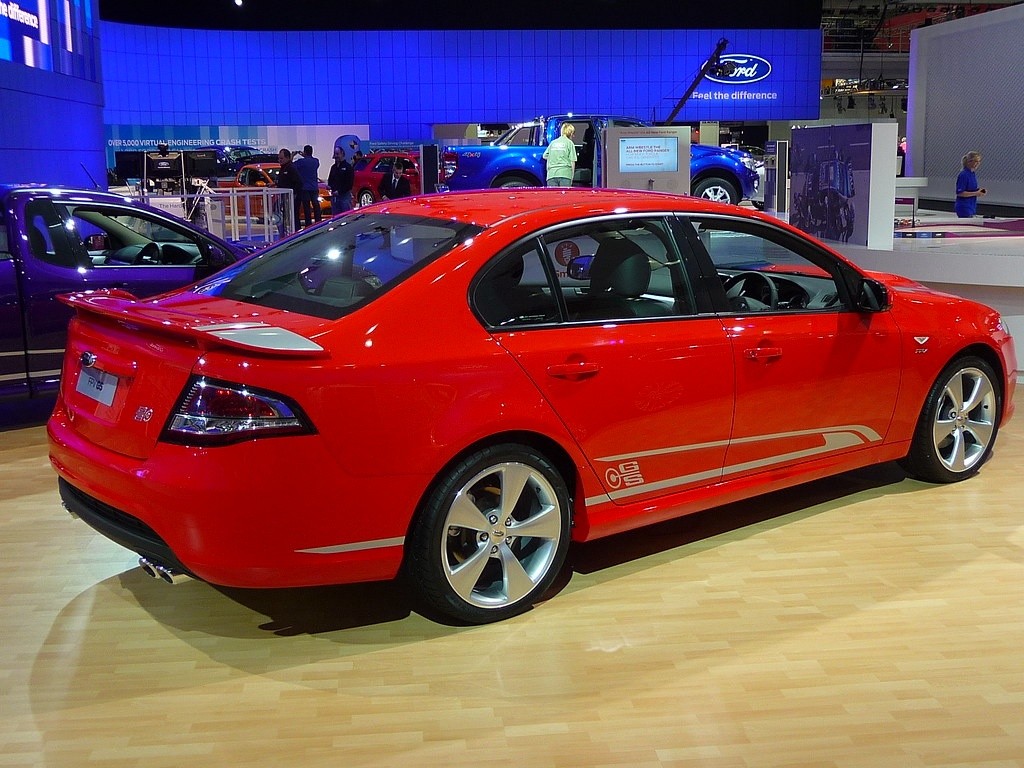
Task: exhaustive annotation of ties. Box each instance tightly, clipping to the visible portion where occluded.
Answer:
[393,179,397,188]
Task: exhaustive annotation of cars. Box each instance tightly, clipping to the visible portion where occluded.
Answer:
[740,146,766,209]
[46,188,1018,622]
[352,151,457,207]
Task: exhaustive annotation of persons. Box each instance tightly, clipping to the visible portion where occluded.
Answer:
[955,151,987,218]
[327,145,355,215]
[352,151,363,167]
[378,163,410,201]
[899,137,906,153]
[294,145,322,228]
[277,149,301,233]
[543,123,577,187]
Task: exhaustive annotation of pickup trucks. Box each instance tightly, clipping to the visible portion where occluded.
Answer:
[214,163,332,224]
[0,184,384,408]
[439,113,760,206]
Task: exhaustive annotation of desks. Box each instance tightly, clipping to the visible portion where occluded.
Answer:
[895,175,929,228]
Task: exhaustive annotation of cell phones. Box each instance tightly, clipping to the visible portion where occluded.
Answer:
[981,189,986,194]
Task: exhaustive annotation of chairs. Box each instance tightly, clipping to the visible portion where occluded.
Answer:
[574,238,674,322]
[482,257,536,329]
[30,225,47,261]
[319,263,380,307]
